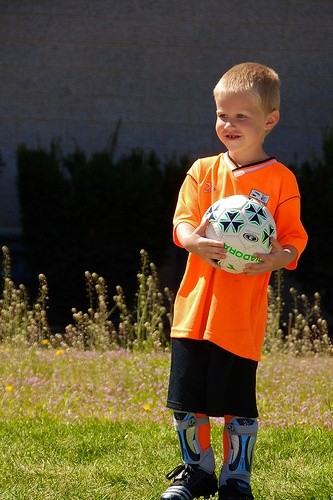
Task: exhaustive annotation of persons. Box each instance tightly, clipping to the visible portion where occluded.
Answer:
[159,62,307,500]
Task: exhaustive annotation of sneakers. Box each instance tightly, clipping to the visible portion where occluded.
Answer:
[160,463,219,500]
[217,478,254,500]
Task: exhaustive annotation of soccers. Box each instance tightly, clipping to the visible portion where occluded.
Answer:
[202,193,278,274]
